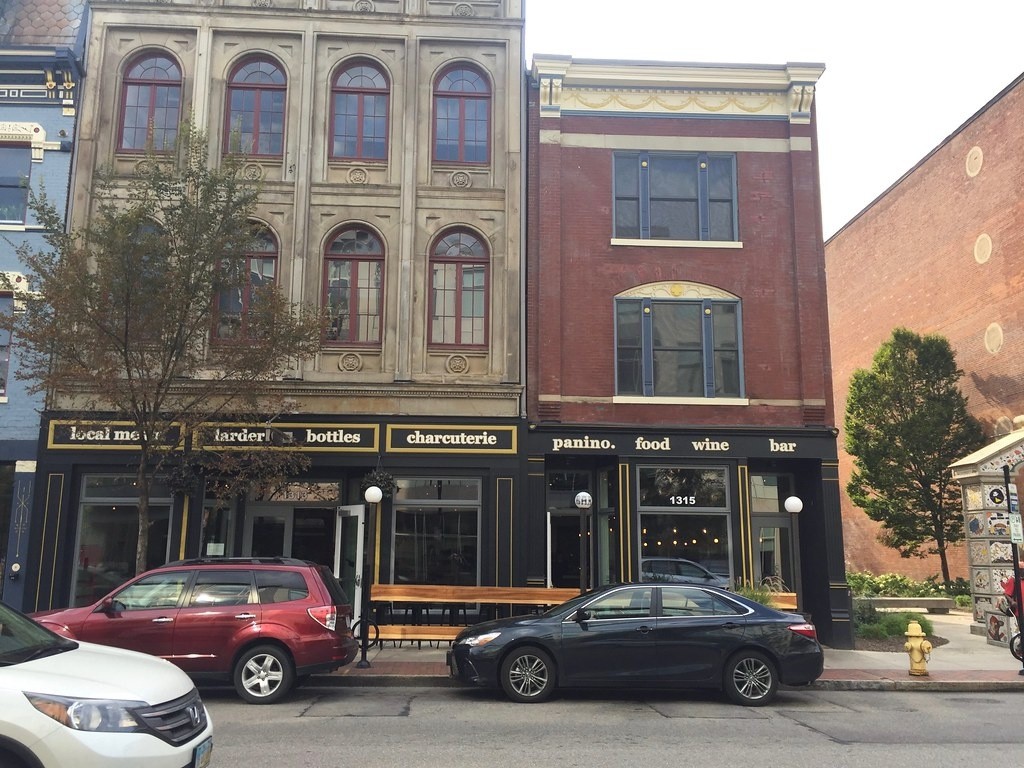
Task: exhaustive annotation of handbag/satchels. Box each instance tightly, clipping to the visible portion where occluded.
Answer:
[998,597,1015,617]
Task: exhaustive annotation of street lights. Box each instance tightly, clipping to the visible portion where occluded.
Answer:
[355,485,383,669]
[785,495,803,611]
[575,491,593,594]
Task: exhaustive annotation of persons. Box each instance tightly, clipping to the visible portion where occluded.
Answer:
[1003,562,1024,625]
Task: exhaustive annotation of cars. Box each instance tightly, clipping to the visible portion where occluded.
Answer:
[74,567,125,606]
[446,582,824,708]
[0,600,215,768]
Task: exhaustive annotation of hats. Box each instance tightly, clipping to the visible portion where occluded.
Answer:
[1019,561,1024,569]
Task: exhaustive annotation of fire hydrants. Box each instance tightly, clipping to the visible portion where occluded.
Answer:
[903,618,933,676]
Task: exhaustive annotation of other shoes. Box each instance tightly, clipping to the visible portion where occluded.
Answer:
[1018,668,1024,675]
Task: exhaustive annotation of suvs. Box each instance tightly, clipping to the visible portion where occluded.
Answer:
[641,555,730,591]
[24,556,361,706]
